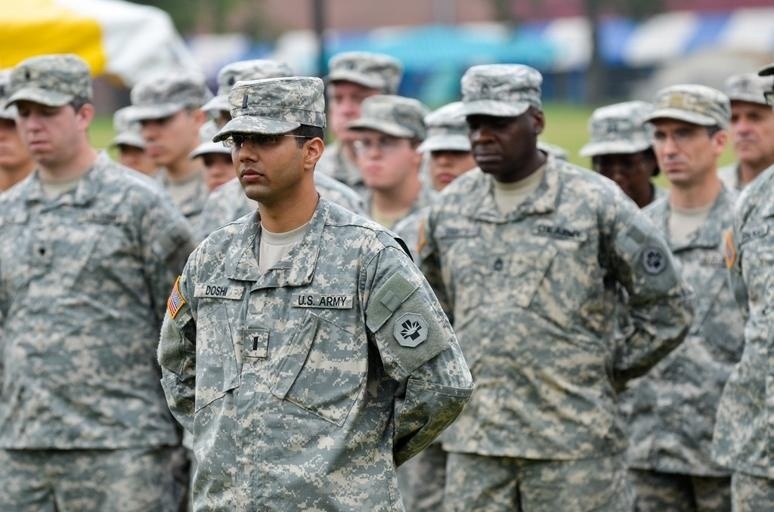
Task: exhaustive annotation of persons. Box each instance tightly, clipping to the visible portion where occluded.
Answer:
[414,62,694,510]
[1,52,199,512]
[707,162,774,512]
[154,76,475,512]
[631,85,743,512]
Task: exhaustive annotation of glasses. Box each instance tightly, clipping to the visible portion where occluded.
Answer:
[221,132,314,148]
[763,90,774,106]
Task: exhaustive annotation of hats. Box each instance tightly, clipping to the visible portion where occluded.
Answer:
[720,74,773,108]
[579,99,653,160]
[211,75,327,144]
[642,84,733,130]
[188,118,232,163]
[415,100,472,156]
[121,70,214,122]
[757,64,774,77]
[0,74,19,120]
[325,51,404,92]
[343,95,430,141]
[5,53,94,110]
[201,58,294,114]
[457,63,545,118]
[109,107,146,151]
